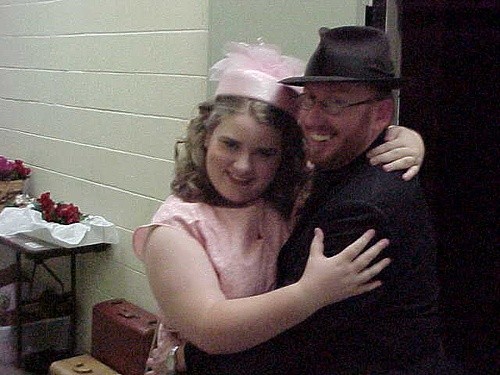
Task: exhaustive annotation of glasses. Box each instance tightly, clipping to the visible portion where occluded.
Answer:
[297,93,392,114]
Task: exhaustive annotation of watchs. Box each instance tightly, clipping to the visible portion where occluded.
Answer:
[164,345,180,375]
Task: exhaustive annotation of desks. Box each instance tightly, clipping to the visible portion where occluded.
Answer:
[0,233,111,368]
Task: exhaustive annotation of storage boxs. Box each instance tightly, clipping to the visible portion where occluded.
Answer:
[0,280,39,313]
[0,316,71,366]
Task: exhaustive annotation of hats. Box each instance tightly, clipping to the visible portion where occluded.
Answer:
[277,26,424,86]
[208,42,307,121]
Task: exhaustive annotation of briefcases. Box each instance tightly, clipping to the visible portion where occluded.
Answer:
[50,355,119,375]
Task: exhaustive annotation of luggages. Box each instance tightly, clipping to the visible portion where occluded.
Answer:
[90,299,163,375]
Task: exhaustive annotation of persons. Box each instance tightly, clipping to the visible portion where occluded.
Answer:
[141,27,441,375]
[131,41,425,375]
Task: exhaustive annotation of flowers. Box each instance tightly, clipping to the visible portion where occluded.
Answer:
[0,155,31,181]
[31,191,90,226]
